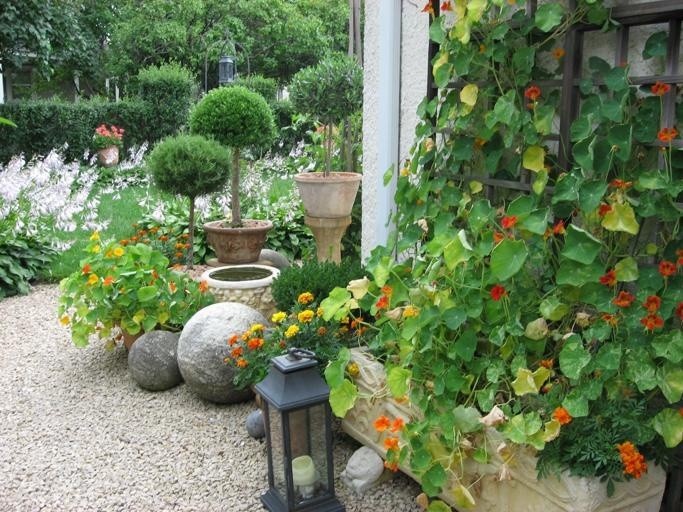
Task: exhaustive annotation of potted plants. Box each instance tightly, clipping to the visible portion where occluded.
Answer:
[189,86,274,264]
[288,52,363,218]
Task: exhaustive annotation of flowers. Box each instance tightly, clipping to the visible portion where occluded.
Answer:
[58,221,212,353]
[224,291,365,388]
[92,124,125,149]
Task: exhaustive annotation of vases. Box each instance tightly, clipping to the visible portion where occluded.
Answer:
[202,264,281,322]
[96,144,119,168]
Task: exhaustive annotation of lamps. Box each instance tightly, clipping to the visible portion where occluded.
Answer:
[254,348,346,510]
